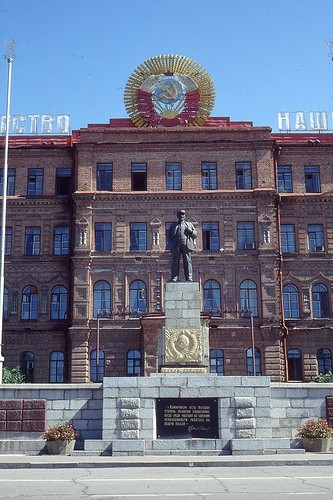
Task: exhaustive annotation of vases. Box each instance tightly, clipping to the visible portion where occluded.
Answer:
[301,438,330,452]
[47,440,75,455]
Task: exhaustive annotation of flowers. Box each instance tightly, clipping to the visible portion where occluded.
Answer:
[43,422,78,447]
[296,418,333,438]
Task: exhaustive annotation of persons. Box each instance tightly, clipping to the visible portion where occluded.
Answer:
[165,210,198,283]
